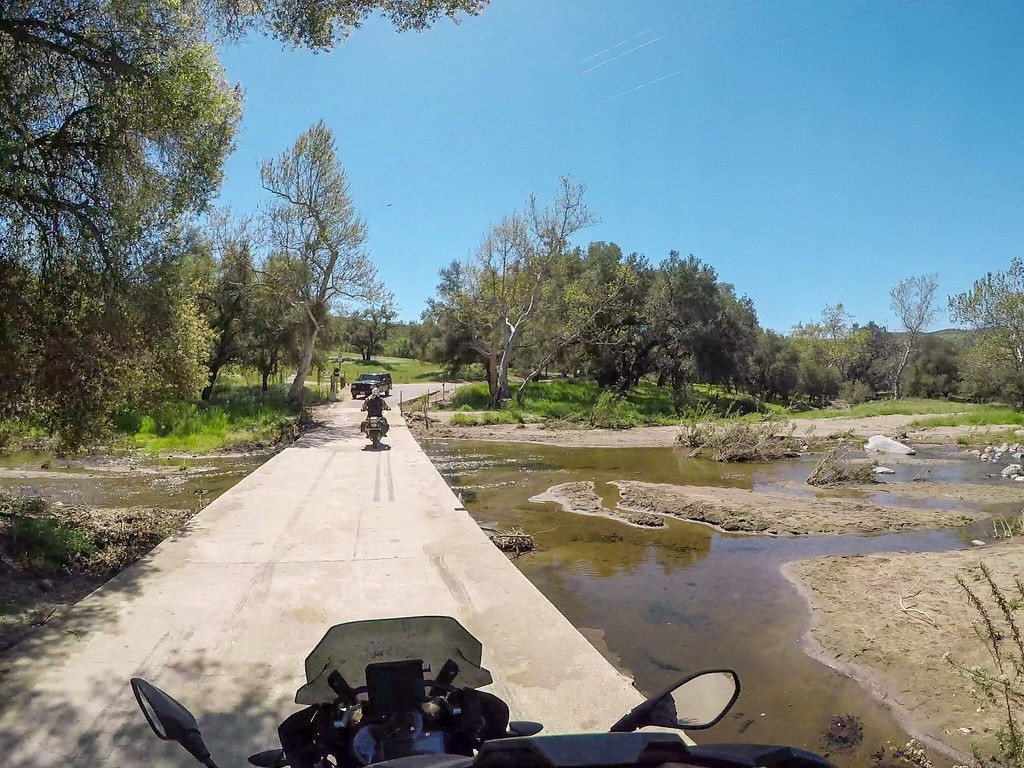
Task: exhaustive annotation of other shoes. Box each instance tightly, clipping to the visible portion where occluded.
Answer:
[365,435,372,439]
[379,432,387,437]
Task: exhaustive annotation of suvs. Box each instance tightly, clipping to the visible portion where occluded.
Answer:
[350,372,393,399]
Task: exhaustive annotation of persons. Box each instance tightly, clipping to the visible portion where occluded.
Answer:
[360,387,391,438]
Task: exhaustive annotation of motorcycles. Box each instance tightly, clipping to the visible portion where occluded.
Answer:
[361,408,391,448]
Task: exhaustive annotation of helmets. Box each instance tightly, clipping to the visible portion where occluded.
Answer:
[372,387,380,394]
